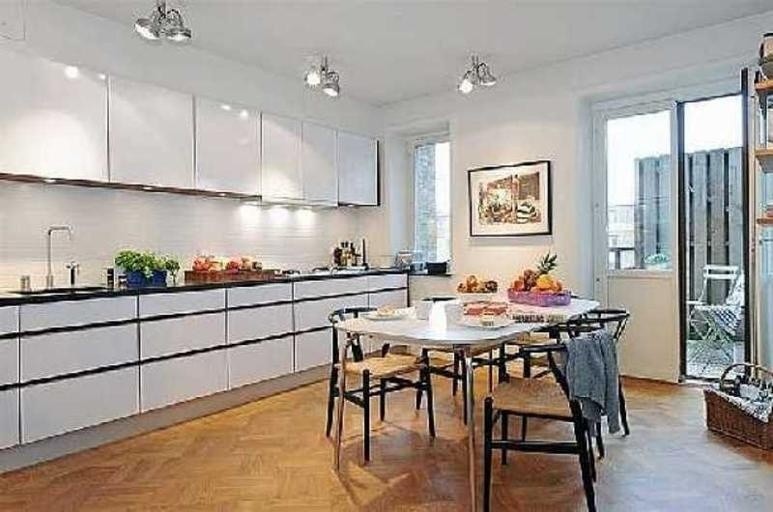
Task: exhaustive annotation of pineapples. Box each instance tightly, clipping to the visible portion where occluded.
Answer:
[523,254,557,291]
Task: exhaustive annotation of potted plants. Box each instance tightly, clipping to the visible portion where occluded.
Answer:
[146,254,177,287]
[115,246,157,285]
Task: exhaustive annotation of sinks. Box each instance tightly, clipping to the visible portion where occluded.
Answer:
[2,285,69,299]
[62,285,125,292]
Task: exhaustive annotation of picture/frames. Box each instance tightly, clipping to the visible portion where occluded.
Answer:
[466,155,551,239]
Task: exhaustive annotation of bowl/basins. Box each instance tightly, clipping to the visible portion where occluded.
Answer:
[459,292,495,305]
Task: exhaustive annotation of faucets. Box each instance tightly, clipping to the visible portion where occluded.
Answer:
[45,224,75,287]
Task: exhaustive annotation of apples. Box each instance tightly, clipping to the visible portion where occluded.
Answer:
[191,259,210,271]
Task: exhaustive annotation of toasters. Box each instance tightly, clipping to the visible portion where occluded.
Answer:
[426,262,447,275]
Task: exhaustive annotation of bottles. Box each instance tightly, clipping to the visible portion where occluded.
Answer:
[334,241,362,267]
[192,259,209,271]
[107,267,113,289]
[117,274,127,290]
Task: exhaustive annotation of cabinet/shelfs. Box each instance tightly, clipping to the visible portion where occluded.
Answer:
[753,78,772,226]
[106,72,195,194]
[336,130,381,208]
[292,273,369,395]
[18,296,140,469]
[226,279,294,406]
[258,112,338,212]
[1,305,21,472]
[195,93,259,202]
[139,284,228,434]
[0,44,109,188]
[367,270,410,357]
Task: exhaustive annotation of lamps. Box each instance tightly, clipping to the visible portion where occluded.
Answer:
[453,52,499,97]
[300,51,343,99]
[132,0,193,45]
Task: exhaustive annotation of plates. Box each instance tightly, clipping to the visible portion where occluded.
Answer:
[363,310,406,321]
[463,316,512,331]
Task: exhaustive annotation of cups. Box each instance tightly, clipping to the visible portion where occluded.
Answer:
[414,301,430,320]
[21,276,29,292]
[507,287,571,306]
[445,304,465,332]
[377,306,394,316]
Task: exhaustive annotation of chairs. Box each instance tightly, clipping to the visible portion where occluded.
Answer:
[322,305,442,466]
[520,302,636,462]
[477,327,611,512]
[411,296,475,428]
[693,269,747,376]
[684,263,739,368]
